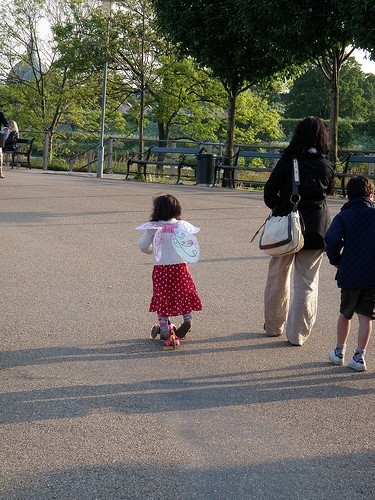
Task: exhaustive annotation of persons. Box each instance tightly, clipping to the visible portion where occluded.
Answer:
[2,120,19,166]
[262,115,333,347]
[137,194,194,348]
[325,176,375,371]
[0,111,10,179]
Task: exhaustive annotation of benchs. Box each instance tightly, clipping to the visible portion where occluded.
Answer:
[125,146,206,185]
[332,153,375,199]
[5,138,36,169]
[211,147,285,189]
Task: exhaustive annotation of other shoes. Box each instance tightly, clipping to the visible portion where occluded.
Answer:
[160,332,170,341]
[175,321,192,338]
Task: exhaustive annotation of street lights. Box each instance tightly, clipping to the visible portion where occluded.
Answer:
[95,17,124,179]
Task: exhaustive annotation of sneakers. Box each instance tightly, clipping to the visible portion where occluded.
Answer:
[329,350,344,365]
[346,356,367,371]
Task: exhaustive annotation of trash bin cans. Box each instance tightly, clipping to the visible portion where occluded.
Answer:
[195,153,215,184]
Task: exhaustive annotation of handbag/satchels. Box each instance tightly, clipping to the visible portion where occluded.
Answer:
[250,210,304,257]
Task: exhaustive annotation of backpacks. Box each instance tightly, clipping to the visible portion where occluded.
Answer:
[3,131,17,151]
